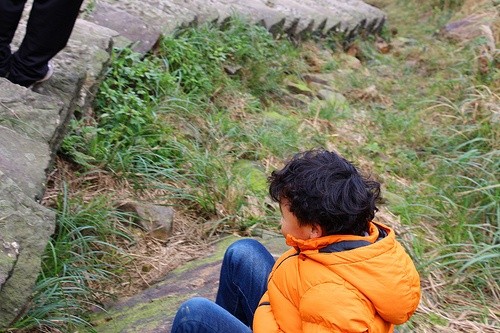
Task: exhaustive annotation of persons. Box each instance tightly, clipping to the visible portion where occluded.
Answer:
[0,0,83,88]
[172,152,421,333]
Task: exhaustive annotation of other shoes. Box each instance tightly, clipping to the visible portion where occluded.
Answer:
[27,63,53,89]
